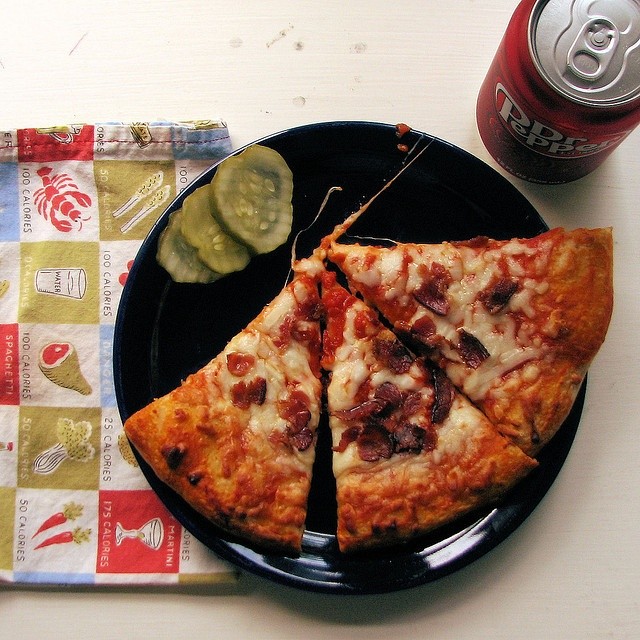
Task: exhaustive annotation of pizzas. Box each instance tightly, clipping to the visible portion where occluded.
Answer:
[320,271,540,557]
[121,256,434,563]
[324,228,614,457]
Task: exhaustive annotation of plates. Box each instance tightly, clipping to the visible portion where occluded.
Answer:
[113,121,587,595]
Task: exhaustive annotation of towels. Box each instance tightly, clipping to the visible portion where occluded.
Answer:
[1,123,244,590]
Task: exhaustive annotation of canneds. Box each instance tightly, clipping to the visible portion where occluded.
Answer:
[476,0,639,188]
